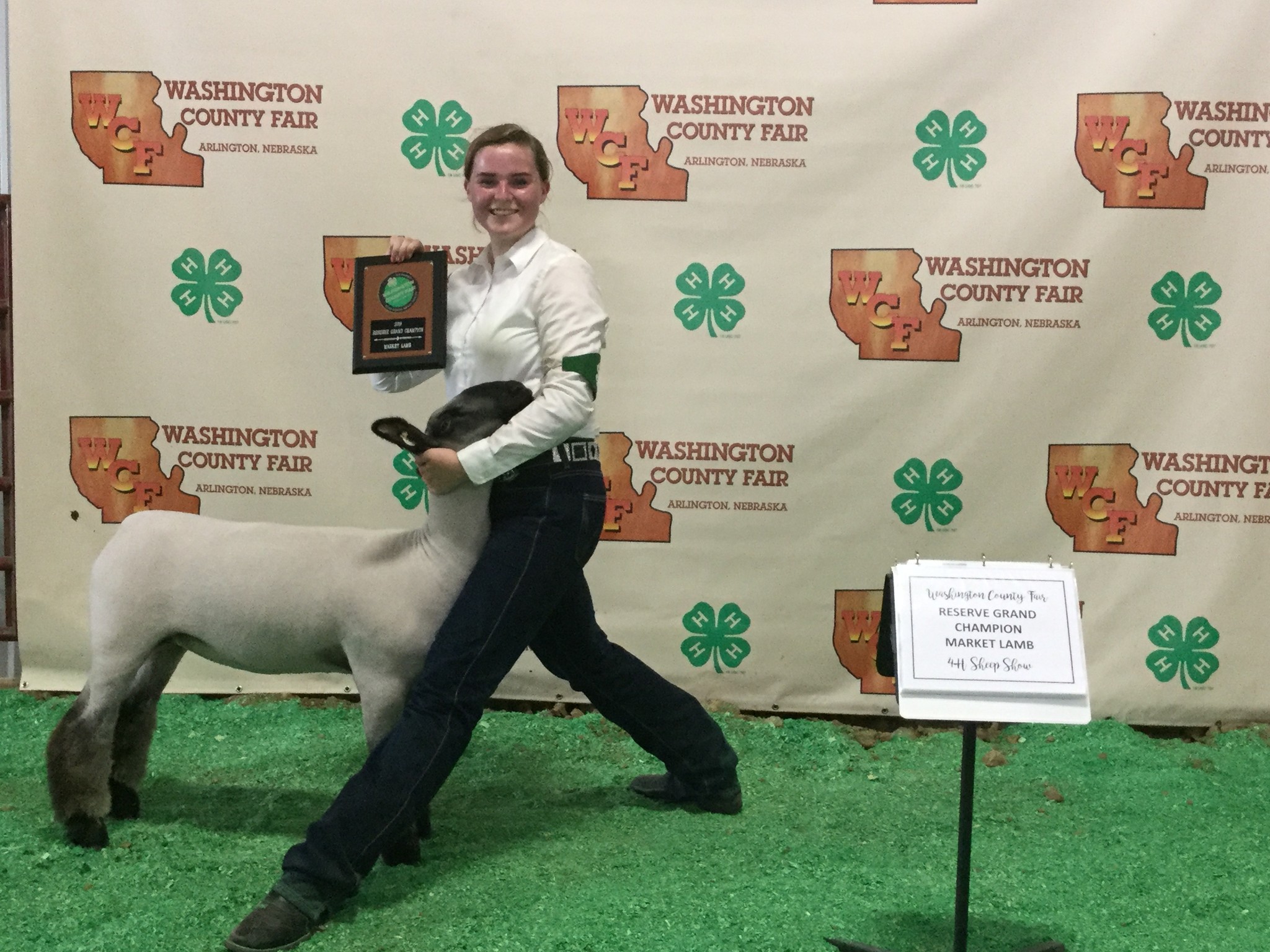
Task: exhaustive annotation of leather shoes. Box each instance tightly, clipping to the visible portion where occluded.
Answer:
[224,890,316,952]
[627,771,742,816]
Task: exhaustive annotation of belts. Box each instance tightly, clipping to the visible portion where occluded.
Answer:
[494,441,600,485]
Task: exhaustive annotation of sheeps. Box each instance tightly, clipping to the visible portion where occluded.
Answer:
[40,375,541,874]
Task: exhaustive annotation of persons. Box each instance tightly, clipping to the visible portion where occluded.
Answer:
[229,123,742,952]
